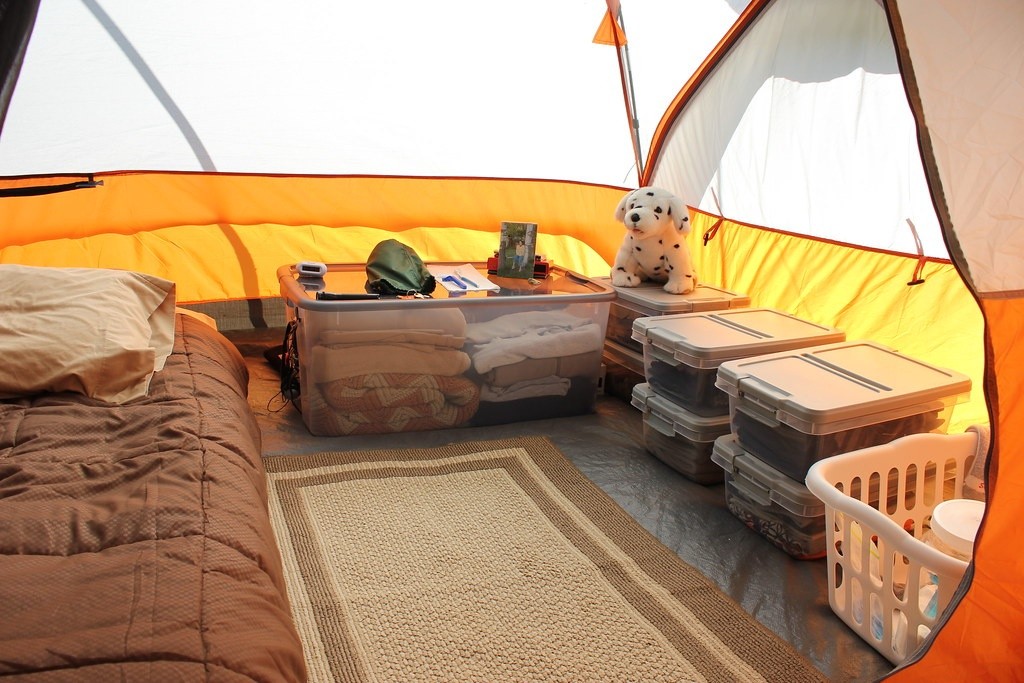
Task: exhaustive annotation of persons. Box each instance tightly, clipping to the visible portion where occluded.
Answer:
[506,234,510,249]
[512,240,525,272]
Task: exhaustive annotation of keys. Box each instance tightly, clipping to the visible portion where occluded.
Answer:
[415,292,429,299]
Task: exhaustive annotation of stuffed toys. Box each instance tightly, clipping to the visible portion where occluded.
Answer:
[608,185,698,293]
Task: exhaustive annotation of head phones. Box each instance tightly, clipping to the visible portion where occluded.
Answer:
[281,321,299,400]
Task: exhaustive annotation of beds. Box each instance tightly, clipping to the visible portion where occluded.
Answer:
[0,301,308,683]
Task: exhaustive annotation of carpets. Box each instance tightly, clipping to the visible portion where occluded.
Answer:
[263,436,830,683]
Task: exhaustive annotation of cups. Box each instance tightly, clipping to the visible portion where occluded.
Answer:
[929,498,986,622]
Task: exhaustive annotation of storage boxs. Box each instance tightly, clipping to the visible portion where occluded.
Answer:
[602,338,645,404]
[630,306,845,417]
[629,383,729,486]
[710,434,958,561]
[275,260,617,436]
[804,431,977,665]
[593,273,751,356]
[714,339,972,484]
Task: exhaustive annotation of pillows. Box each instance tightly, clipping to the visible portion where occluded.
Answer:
[0,263,176,405]
